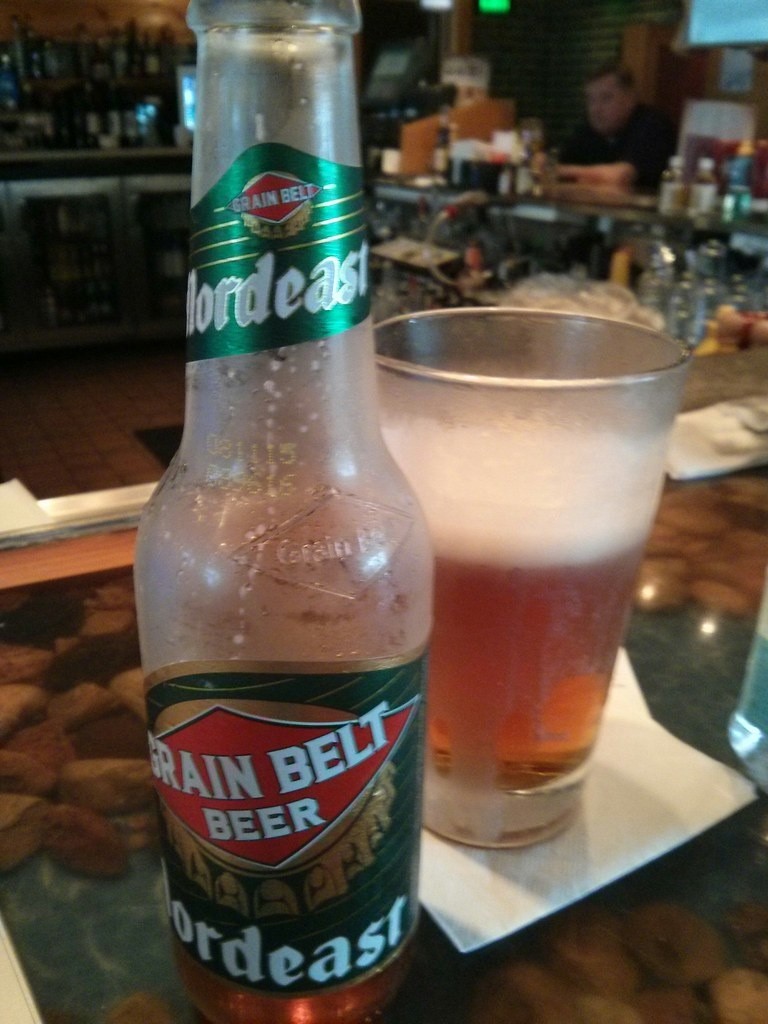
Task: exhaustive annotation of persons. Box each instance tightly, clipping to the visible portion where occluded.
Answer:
[553,61,678,187]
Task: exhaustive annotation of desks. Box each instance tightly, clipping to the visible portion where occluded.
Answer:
[0,461,768,1022]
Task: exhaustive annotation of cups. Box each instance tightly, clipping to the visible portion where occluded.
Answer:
[370,306,694,850]
[520,118,543,164]
[535,150,558,199]
[727,572,768,794]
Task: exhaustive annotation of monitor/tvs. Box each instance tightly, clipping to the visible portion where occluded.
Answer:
[175,63,197,142]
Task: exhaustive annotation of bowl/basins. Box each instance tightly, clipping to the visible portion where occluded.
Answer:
[448,157,517,198]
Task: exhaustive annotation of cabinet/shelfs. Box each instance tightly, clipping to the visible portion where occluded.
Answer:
[0,174,192,351]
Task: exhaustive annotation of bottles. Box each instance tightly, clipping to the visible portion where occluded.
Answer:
[687,156,718,221]
[372,261,422,326]
[104,19,194,150]
[135,0,434,1024]
[657,157,687,217]
[612,225,747,349]
[722,141,754,222]
[42,34,103,151]
[0,17,43,150]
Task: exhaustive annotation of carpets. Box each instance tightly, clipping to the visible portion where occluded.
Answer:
[135,423,184,468]
